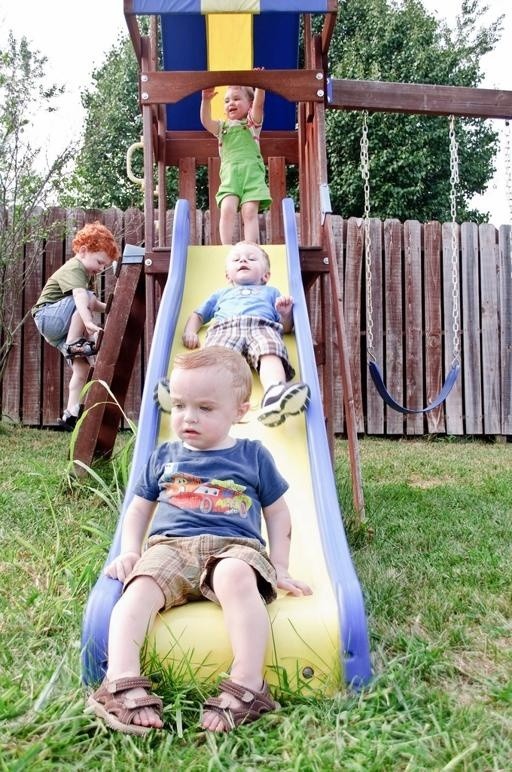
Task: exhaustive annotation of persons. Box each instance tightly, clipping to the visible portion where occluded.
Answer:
[29,218,121,430]
[84,345,315,738]
[152,240,312,428]
[200,66,273,246]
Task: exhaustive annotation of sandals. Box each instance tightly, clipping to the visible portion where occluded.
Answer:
[56,409,78,430]
[86,675,165,738]
[62,337,99,359]
[198,678,282,733]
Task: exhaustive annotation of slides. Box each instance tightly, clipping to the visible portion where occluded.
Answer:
[81,199,370,701]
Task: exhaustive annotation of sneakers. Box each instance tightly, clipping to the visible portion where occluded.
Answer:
[256,381,311,428]
[153,377,173,413]
